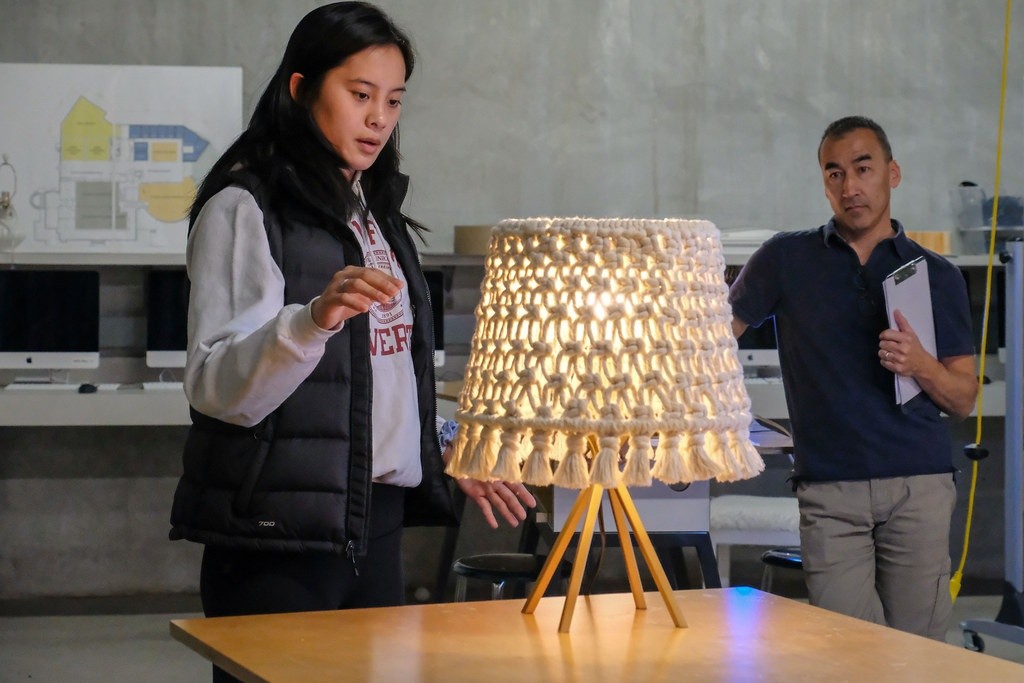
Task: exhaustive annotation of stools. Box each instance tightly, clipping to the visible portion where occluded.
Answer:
[710,495,800,588]
[449,553,572,602]
[760,547,803,593]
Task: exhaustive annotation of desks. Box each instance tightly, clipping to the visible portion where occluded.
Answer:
[170,586,1024,683]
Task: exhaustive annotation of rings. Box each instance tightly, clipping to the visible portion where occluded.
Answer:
[885,351,890,361]
[342,278,349,292]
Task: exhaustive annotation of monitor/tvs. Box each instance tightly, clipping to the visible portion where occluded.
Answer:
[954,265,1007,356]
[144,270,192,367]
[0,269,101,384]
[421,269,444,366]
[723,265,780,366]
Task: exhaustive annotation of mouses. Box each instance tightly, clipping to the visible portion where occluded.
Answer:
[977,374,992,384]
[79,383,97,393]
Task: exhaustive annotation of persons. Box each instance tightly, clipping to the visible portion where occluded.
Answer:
[726,115,982,640]
[166,0,541,683]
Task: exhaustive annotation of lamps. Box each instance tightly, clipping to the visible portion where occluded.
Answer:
[444,214,766,635]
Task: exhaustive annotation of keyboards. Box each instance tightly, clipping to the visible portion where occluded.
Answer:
[5,383,121,391]
[743,376,782,384]
[143,382,182,391]
